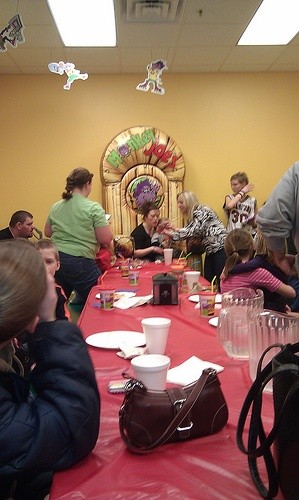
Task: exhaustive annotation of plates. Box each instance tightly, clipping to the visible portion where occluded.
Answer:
[85,331,146,349]
[117,266,141,269]
[208,316,218,327]
[189,294,223,303]
[96,291,136,299]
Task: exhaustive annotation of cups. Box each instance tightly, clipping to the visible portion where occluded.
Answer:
[131,353,170,391]
[141,317,171,354]
[247,308,298,395]
[217,287,263,360]
[172,258,187,277]
[199,291,217,317]
[119,259,130,277]
[185,271,201,290]
[98,286,116,311]
[164,249,173,266]
[127,269,140,286]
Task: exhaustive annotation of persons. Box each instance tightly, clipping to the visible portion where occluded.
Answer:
[284,253,299,315]
[220,228,298,314]
[152,216,183,263]
[221,232,288,313]
[130,201,188,266]
[162,192,229,293]
[2,238,103,500]
[255,160,299,278]
[13,239,75,377]
[2,211,37,241]
[71,236,125,306]
[45,167,114,305]
[223,172,259,238]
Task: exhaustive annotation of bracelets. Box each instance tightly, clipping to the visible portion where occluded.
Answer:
[238,190,246,197]
[153,246,159,254]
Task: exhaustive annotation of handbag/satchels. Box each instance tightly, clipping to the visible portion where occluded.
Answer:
[119,369,228,451]
[236,342,299,500]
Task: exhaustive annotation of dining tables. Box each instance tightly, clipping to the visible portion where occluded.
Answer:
[49,259,299,499]
